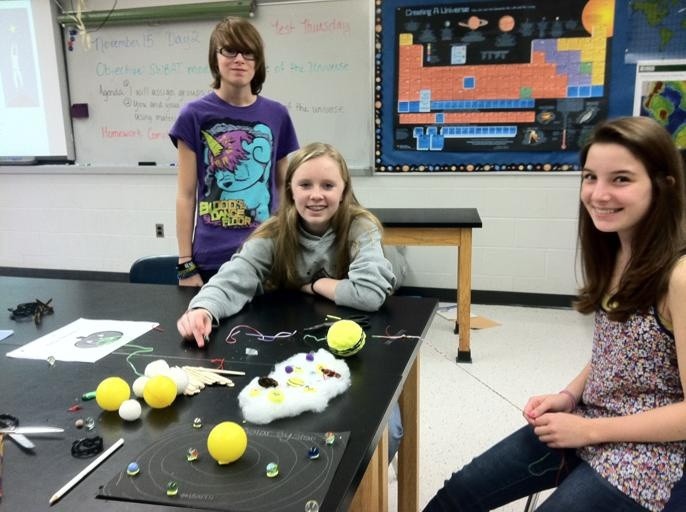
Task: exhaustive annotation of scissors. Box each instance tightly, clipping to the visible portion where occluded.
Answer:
[304,314,371,331]
[0,414,65,449]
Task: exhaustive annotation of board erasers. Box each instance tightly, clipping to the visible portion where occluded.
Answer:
[138,161,157,166]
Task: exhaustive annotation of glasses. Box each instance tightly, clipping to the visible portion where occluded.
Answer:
[217,45,258,60]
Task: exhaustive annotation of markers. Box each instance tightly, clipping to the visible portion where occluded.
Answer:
[65,162,92,167]
[170,164,178,168]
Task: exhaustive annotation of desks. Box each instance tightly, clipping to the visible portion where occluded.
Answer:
[360,205,487,363]
[0,273,440,512]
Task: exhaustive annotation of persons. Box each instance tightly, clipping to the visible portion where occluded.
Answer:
[417,115,686,511]
[168,12,300,289]
[176,141,403,469]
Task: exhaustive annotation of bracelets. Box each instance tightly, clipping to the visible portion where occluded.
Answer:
[178,259,195,270]
[179,270,195,278]
[560,389,577,411]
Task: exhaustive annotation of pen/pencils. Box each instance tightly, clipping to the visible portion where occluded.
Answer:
[49,438,125,506]
[81,390,97,400]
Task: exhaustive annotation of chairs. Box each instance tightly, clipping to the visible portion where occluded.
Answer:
[126,252,186,284]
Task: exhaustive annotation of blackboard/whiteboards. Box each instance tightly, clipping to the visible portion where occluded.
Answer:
[0,0,377,177]
